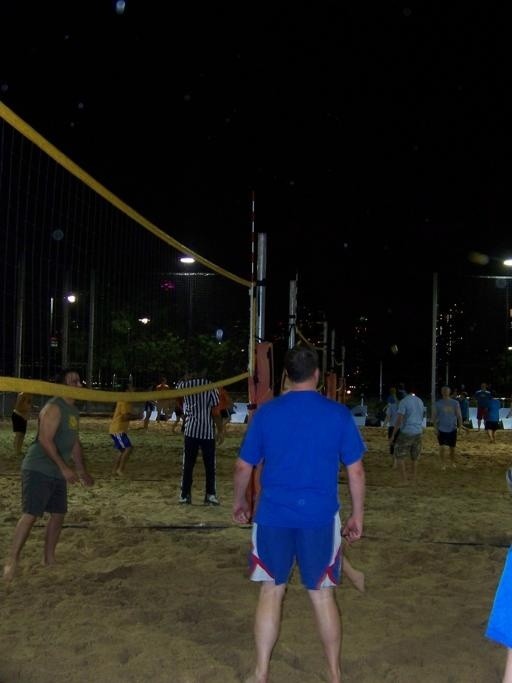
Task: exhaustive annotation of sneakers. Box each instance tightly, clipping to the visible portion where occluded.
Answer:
[178,493,191,503]
[206,493,220,504]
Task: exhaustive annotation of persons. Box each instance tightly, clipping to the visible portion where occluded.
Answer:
[2,371,95,591]
[142,377,185,434]
[485,545,511,682]
[12,391,34,457]
[342,557,366,591]
[451,382,499,444]
[230,350,365,683]
[433,385,462,471]
[108,384,144,479]
[214,388,229,435]
[384,384,426,488]
[176,362,220,507]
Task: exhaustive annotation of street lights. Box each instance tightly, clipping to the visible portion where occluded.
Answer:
[180,256,199,380]
[61,293,79,367]
[131,311,153,386]
[432,253,512,430]
